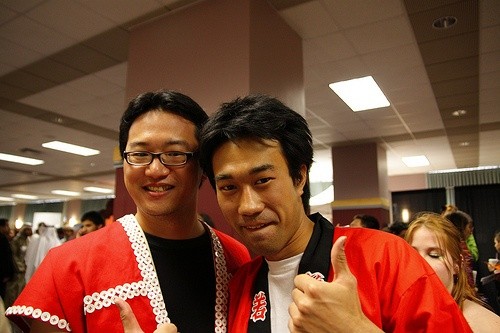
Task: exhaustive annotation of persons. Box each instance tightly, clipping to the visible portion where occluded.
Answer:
[100,198,114,226]
[26,222,46,283]
[402,212,500,333]
[75,211,105,239]
[388,222,409,240]
[487,234,500,274]
[441,205,477,261]
[10,222,33,304]
[63,227,75,242]
[446,213,472,289]
[33,226,63,272]
[348,214,380,231]
[3,88,252,333]
[0,219,19,309]
[197,94,474,333]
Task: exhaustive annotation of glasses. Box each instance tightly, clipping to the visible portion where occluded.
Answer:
[122,150,195,166]
